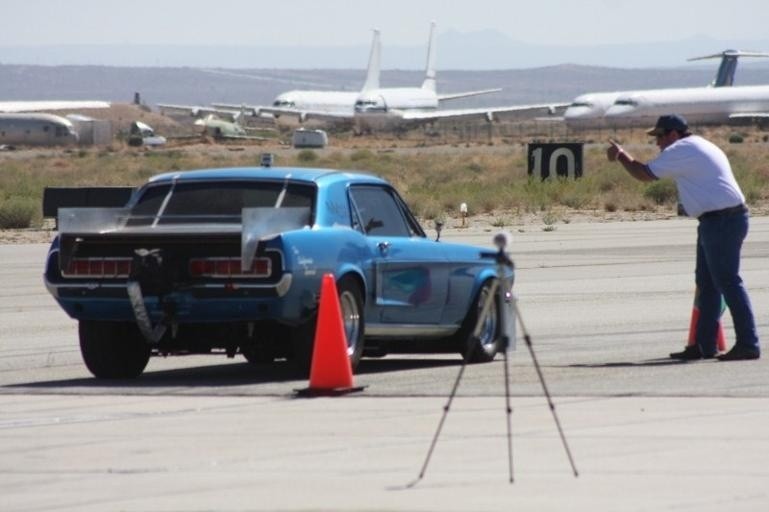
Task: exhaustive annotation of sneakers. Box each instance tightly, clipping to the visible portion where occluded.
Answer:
[669,345,718,360]
[717,346,760,361]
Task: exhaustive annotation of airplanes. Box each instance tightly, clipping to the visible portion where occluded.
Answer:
[562,49,769,131]
[0,100,111,147]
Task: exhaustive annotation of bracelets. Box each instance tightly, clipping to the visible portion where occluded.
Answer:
[615,149,623,159]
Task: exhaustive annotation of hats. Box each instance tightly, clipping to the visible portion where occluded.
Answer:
[647,114,688,137]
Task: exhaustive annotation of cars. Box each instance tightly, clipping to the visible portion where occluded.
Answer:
[41,166,517,381]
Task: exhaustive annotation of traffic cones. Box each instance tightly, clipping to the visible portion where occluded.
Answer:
[685,286,728,355]
[291,272,370,396]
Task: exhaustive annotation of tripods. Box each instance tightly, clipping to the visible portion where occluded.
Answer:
[383,264,577,483]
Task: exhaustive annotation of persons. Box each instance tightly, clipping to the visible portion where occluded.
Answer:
[608,114,760,361]
[365,217,383,231]
